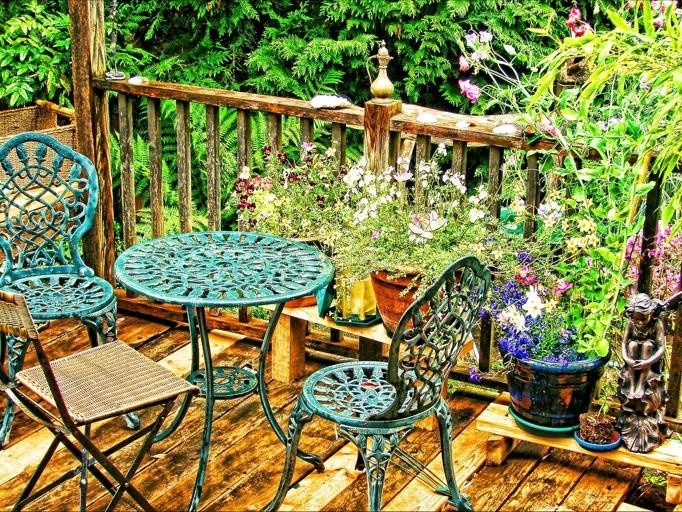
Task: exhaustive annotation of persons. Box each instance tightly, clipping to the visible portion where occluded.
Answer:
[621,292,668,401]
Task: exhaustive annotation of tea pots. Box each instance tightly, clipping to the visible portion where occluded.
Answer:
[366,37,394,106]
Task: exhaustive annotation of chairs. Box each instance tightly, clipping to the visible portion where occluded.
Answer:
[0,131,140,449]
[262,257,491,512]
[0,290,200,512]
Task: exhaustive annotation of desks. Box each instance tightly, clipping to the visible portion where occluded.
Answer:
[115,230,335,511]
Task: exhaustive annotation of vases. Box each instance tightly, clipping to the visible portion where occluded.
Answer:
[496,334,612,436]
